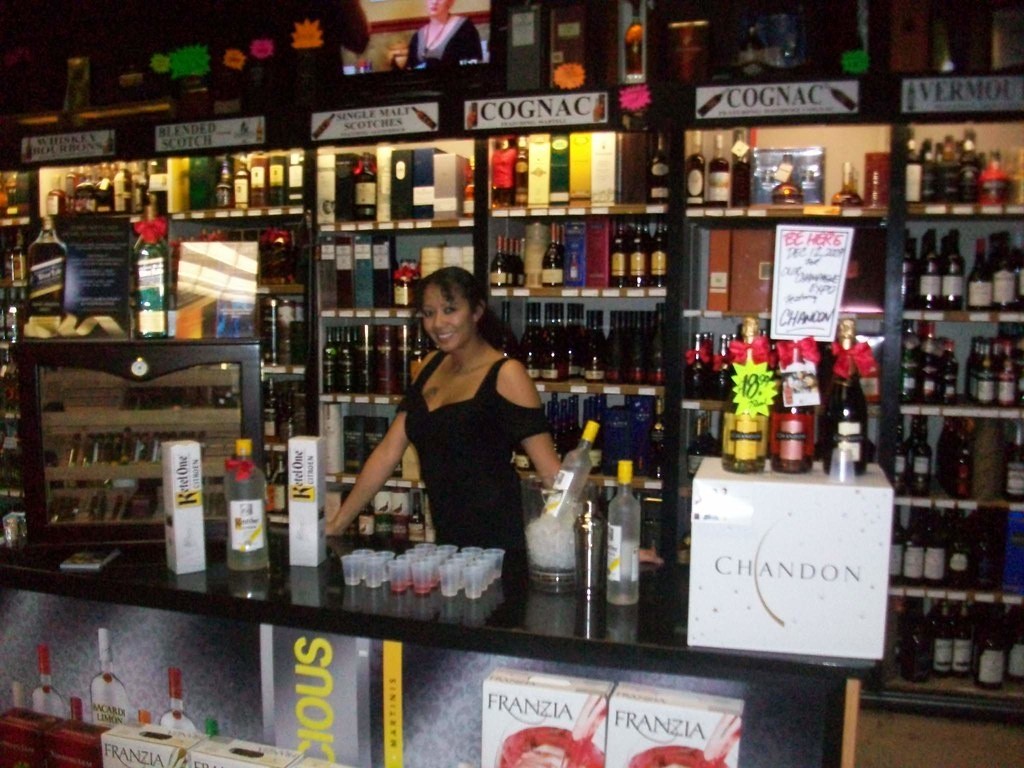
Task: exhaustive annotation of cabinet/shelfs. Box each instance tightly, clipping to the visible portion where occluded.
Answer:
[0,69,1024,727]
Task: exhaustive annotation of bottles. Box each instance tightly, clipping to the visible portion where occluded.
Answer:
[312,114,335,139]
[160,667,195,731]
[25,216,67,321]
[819,319,869,476]
[825,83,857,110]
[31,642,65,720]
[488,129,1024,691]
[768,341,817,474]
[70,696,83,720]
[90,628,128,730]
[132,205,168,339]
[540,420,600,523]
[138,709,153,724]
[0,156,307,518]
[412,107,436,130]
[313,326,438,541]
[697,88,728,117]
[224,439,270,571]
[257,117,262,143]
[12,681,26,708]
[720,317,775,475]
[606,460,640,604]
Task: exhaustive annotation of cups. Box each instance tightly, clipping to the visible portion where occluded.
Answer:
[340,542,506,600]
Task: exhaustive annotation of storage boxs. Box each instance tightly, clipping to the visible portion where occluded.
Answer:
[855,334,885,402]
[863,153,889,208]
[402,444,421,479]
[506,0,661,92]
[0,706,356,768]
[288,436,327,567]
[606,393,657,473]
[566,216,617,287]
[688,457,894,660]
[166,156,219,213]
[1003,510,1024,595]
[482,668,746,768]
[161,440,207,575]
[325,491,341,523]
[316,143,470,220]
[322,234,397,308]
[709,227,775,312]
[888,1,928,73]
[232,222,307,294]
[751,148,823,203]
[528,131,649,207]
[16,171,29,215]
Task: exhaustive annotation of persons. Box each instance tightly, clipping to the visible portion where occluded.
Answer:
[323,266,665,565]
[385,0,483,70]
